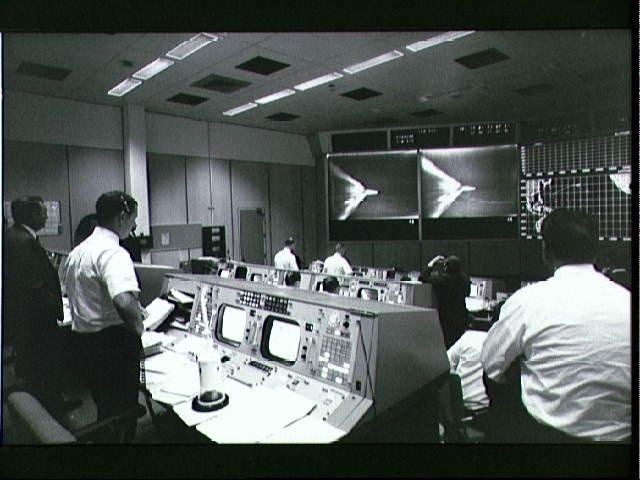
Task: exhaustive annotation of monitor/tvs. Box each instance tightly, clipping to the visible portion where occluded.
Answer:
[214,303,247,349]
[260,315,301,367]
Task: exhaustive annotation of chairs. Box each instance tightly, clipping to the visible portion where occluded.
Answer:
[427,371,500,441]
[4,379,148,442]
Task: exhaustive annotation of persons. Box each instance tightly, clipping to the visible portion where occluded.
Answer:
[444,296,507,433]
[65,190,146,445]
[5,194,84,414]
[324,242,354,276]
[273,235,300,270]
[416,253,472,344]
[481,206,635,446]
[73,209,98,240]
